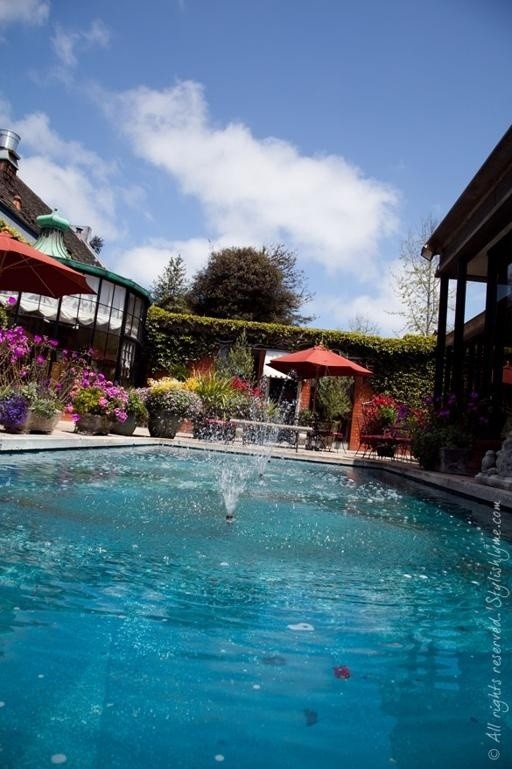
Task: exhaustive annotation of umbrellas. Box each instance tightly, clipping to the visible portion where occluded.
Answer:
[268,345,375,415]
[1,232,100,302]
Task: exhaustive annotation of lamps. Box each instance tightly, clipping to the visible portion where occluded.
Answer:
[420,247,435,260]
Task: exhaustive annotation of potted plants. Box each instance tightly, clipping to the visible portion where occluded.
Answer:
[111,377,202,439]
[28,399,63,432]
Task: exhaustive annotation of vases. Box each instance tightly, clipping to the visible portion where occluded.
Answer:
[375,443,396,458]
[422,447,464,472]
[74,413,113,436]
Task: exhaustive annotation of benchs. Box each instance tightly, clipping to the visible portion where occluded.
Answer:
[229,419,314,454]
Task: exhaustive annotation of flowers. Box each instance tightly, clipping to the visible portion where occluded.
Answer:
[65,370,128,423]
[357,391,490,447]
